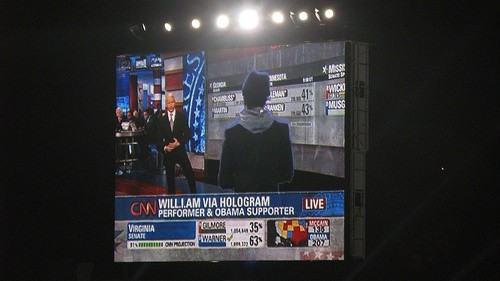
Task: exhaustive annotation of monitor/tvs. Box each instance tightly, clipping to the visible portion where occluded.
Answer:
[112,39,351,263]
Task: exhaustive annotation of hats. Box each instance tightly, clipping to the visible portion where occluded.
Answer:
[242,70,271,100]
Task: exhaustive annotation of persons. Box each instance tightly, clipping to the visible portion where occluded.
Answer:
[115,103,163,176]
[216,71,296,193]
[154,92,198,194]
